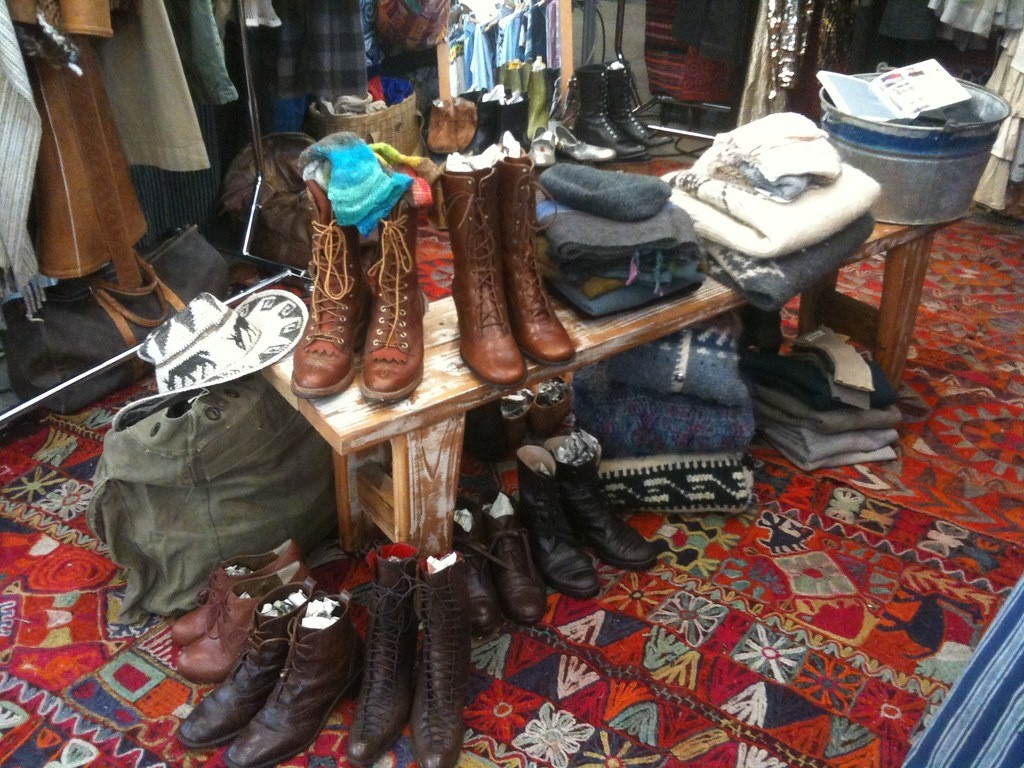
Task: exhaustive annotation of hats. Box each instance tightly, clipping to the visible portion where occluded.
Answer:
[113,286,309,430]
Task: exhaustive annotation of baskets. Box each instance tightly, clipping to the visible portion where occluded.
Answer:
[310,90,419,157]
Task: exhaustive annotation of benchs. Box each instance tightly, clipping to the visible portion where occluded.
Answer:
[262,216,971,554]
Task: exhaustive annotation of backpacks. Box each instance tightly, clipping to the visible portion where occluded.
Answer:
[92,403,336,615]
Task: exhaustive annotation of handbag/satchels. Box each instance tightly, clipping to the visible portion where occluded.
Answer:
[3,220,227,416]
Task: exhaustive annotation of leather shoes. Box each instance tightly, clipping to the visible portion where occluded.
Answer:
[530,125,618,165]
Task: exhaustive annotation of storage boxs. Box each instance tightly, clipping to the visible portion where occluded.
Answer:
[644,1,734,106]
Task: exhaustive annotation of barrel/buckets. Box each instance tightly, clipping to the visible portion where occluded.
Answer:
[818,61,1011,224]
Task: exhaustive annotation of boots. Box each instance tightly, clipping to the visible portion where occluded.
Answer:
[440,154,580,389]
[576,59,675,160]
[288,153,428,404]
[424,56,558,155]
[168,432,661,767]
